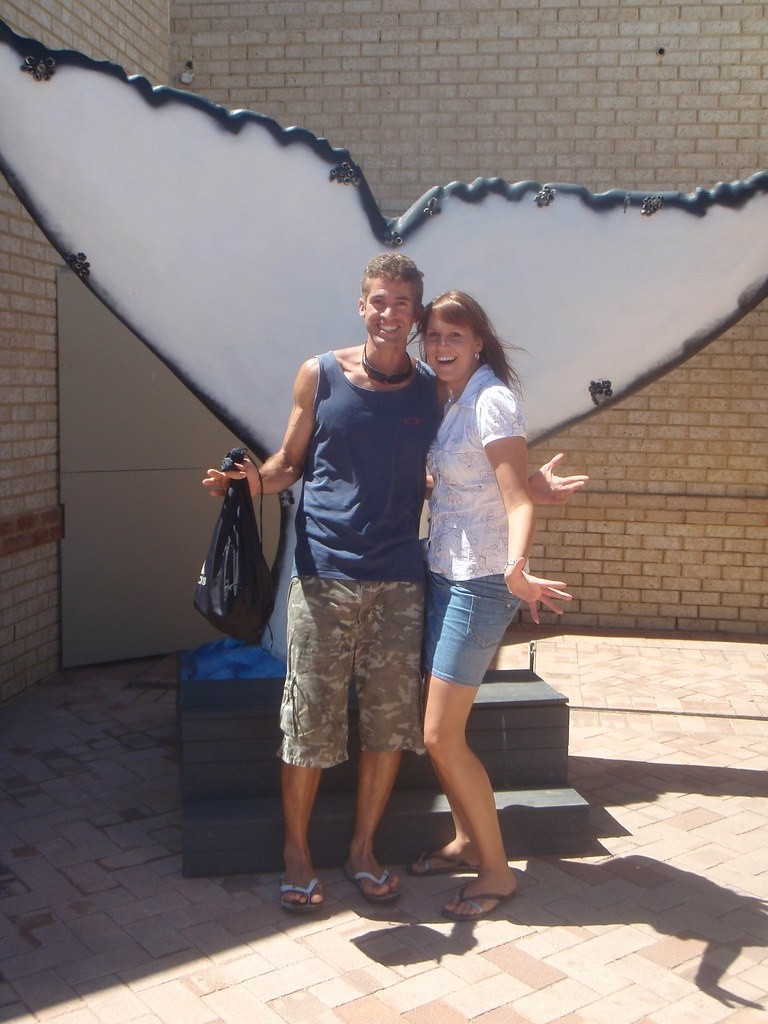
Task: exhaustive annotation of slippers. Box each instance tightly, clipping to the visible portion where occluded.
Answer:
[279,872,324,913]
[407,848,479,877]
[441,885,516,921]
[342,862,401,903]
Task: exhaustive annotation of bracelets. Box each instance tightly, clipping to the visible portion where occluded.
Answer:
[504,560,526,594]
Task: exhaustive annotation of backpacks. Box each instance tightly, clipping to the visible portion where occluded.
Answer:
[193,447,277,652]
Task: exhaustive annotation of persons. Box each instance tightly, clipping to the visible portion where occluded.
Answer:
[202,253,589,919]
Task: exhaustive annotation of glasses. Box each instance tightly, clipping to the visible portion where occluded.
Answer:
[361,340,414,385]
